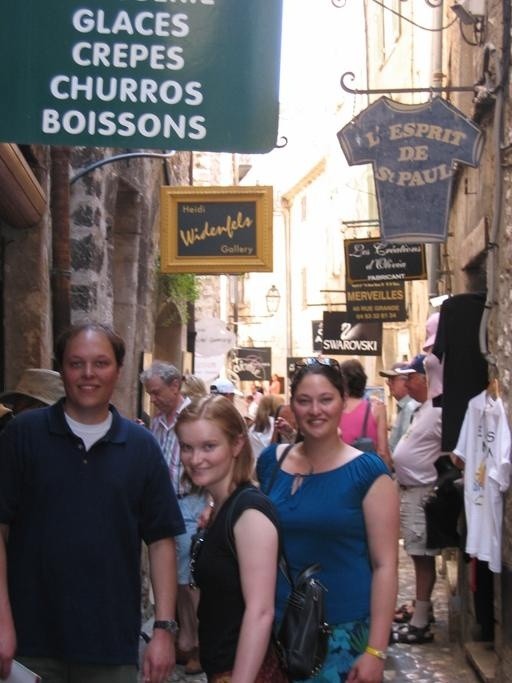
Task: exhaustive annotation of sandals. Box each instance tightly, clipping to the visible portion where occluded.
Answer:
[393,597,435,622]
[389,625,434,644]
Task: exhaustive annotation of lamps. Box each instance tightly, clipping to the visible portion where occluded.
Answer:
[229,285,281,317]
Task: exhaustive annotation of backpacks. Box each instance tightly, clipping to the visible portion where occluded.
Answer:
[224,486,330,681]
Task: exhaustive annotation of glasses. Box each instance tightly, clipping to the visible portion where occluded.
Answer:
[187,536,206,587]
[293,356,340,372]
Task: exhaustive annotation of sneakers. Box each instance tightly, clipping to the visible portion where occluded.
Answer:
[174,630,197,664]
[184,652,204,675]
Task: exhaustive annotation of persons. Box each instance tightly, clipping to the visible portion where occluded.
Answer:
[1,321,512,681]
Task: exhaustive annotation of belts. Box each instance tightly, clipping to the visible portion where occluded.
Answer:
[396,480,436,491]
[175,492,189,499]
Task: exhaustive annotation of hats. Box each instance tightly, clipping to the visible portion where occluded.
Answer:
[211,378,243,398]
[1,368,67,408]
[421,313,439,351]
[379,362,409,376]
[395,352,426,374]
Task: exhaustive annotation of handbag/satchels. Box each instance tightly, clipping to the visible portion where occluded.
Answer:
[421,493,466,549]
[348,438,375,453]
